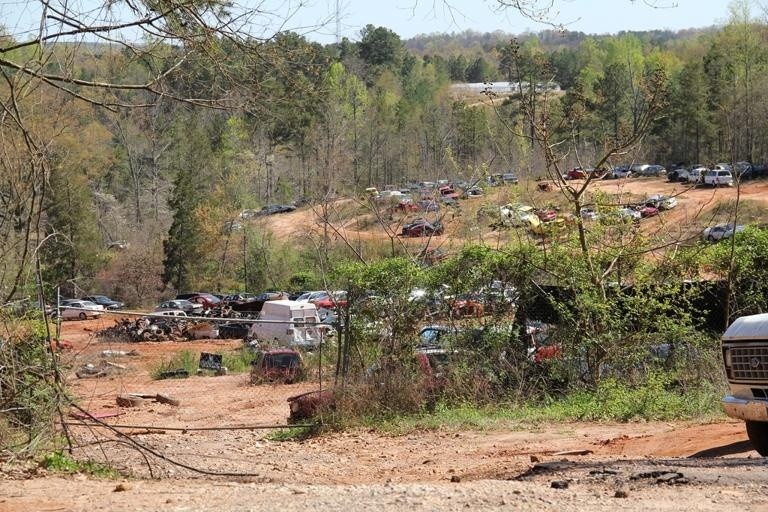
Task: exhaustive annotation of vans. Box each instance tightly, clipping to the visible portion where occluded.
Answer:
[248,300,321,351]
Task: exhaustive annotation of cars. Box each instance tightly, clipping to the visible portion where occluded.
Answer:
[251,348,304,384]
[402,218,443,237]
[720,312,768,457]
[562,162,768,187]
[365,173,518,212]
[500,194,678,234]
[224,205,296,232]
[152,291,349,324]
[51,295,125,321]
[703,222,745,241]
